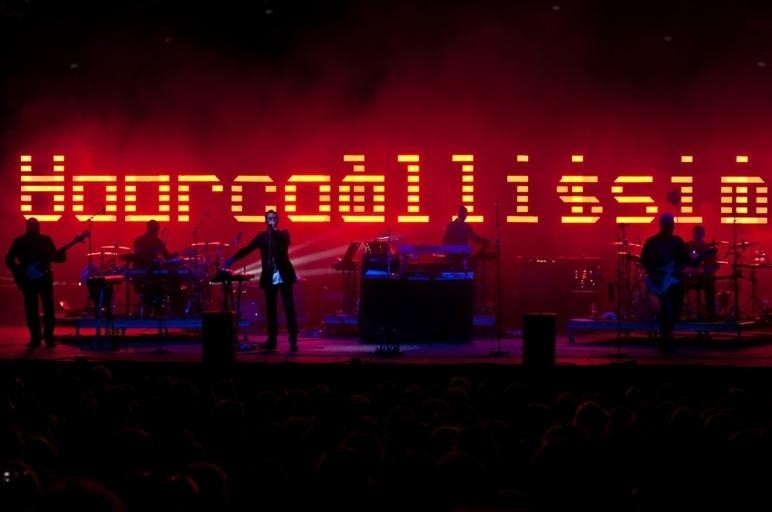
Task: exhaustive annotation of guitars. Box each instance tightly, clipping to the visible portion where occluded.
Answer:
[644,248,719,296]
[11,232,90,294]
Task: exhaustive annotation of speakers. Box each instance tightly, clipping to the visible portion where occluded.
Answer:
[522,312,556,364]
[203,310,237,360]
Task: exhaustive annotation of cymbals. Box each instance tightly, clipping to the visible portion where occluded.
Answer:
[611,242,639,247]
[750,263,772,268]
[616,252,641,259]
[710,240,729,244]
[713,259,728,264]
[733,241,748,246]
[99,245,131,252]
[191,242,206,246]
[207,242,230,248]
[121,253,142,263]
[86,253,118,257]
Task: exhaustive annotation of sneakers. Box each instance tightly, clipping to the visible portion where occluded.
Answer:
[259,342,277,349]
[291,344,298,352]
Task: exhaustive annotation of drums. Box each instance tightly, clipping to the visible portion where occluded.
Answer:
[143,258,161,272]
[164,258,186,272]
[570,269,596,288]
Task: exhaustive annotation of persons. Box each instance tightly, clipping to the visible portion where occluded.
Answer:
[0,360,769,511]
[443,205,490,271]
[685,224,717,315]
[225,210,298,352]
[133,219,180,312]
[640,212,704,354]
[5,218,67,348]
